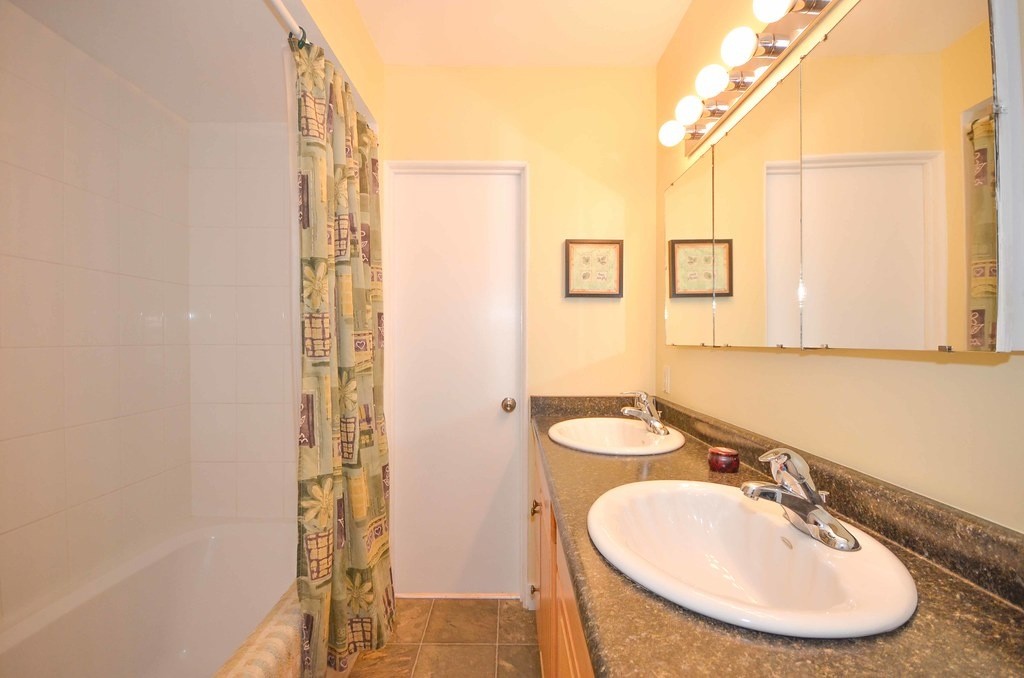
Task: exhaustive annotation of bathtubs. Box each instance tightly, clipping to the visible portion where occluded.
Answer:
[0,515,303,678]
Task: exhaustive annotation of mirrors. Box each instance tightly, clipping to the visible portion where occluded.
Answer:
[664,0,1023,355]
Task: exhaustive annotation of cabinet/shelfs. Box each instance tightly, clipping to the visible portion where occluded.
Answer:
[532,431,594,678]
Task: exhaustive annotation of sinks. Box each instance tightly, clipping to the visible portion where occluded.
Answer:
[548,418,687,456]
[587,479,918,638]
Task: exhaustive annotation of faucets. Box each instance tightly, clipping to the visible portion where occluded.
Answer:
[619,390,668,435]
[741,447,859,551]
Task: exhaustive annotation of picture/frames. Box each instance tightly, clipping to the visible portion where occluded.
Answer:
[564,239,623,298]
[669,239,733,298]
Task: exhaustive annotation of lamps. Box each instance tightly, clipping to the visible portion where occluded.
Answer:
[658,0,833,147]
